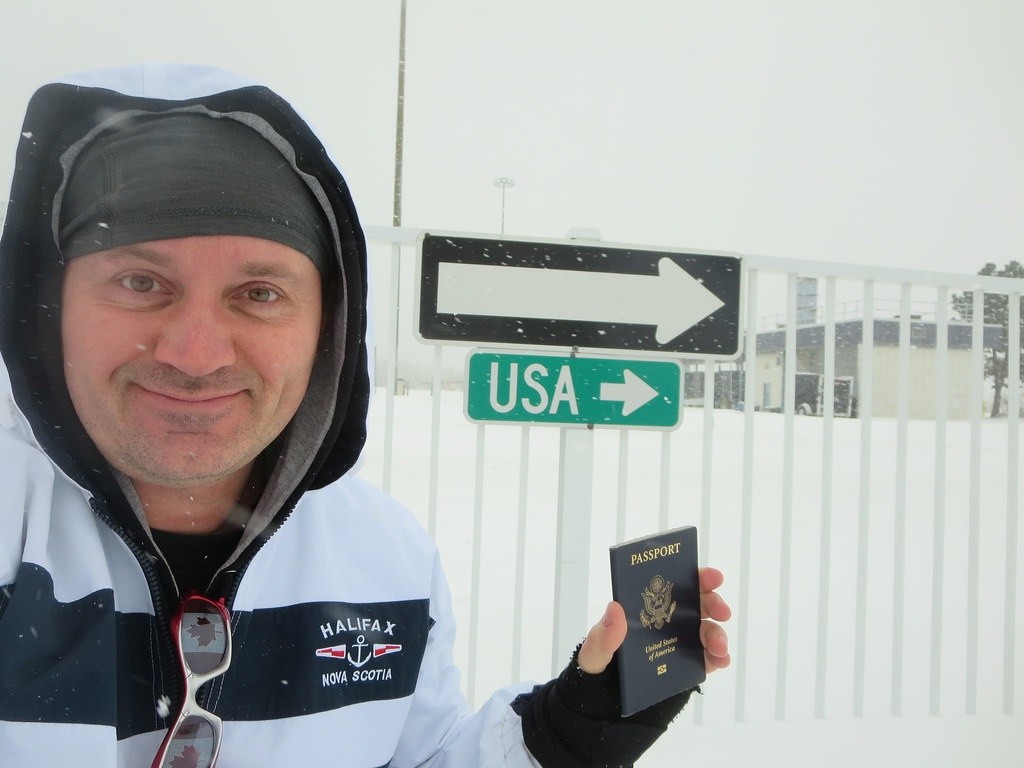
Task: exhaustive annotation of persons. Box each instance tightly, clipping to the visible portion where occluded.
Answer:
[1,62,732,768]
